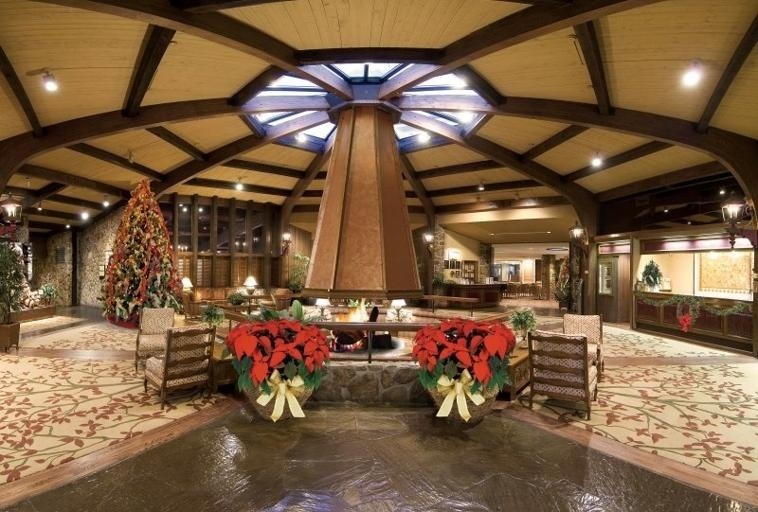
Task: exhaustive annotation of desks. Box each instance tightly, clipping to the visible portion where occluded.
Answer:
[500,342,530,401]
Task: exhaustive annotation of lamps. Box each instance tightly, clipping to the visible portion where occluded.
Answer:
[569,221,582,237]
[235,176,243,191]
[721,191,745,220]
[391,299,407,320]
[315,298,331,320]
[479,181,484,190]
[243,276,258,302]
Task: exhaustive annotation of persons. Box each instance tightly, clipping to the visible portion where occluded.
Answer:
[326,306,379,353]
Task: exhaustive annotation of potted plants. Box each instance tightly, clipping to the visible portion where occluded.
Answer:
[0,242,27,352]
[510,307,536,339]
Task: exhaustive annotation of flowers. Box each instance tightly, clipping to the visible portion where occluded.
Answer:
[412,317,515,422]
[222,322,329,422]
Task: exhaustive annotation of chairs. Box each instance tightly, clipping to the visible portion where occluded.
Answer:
[135,307,174,371]
[520,330,598,420]
[505,280,542,300]
[182,290,207,321]
[563,314,604,383]
[144,324,216,409]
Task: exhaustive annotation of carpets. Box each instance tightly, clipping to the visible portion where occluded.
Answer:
[505,314,757,507]
[0,315,241,511]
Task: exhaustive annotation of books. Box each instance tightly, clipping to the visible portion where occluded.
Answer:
[464,263,475,285]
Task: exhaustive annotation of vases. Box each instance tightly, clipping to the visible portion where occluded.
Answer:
[424,386,499,426]
[243,370,312,422]
